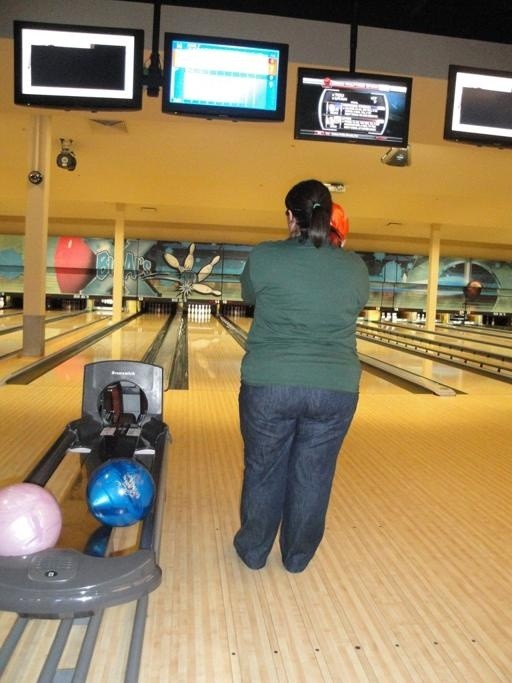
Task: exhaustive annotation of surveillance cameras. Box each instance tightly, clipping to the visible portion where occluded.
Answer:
[57,152,77,170]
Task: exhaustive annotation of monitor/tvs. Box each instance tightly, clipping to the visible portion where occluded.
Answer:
[162,32,288,121]
[294,67,412,148]
[444,64,512,147]
[13,20,144,110]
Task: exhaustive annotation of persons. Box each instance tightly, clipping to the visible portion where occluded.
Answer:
[233,180,370,573]
